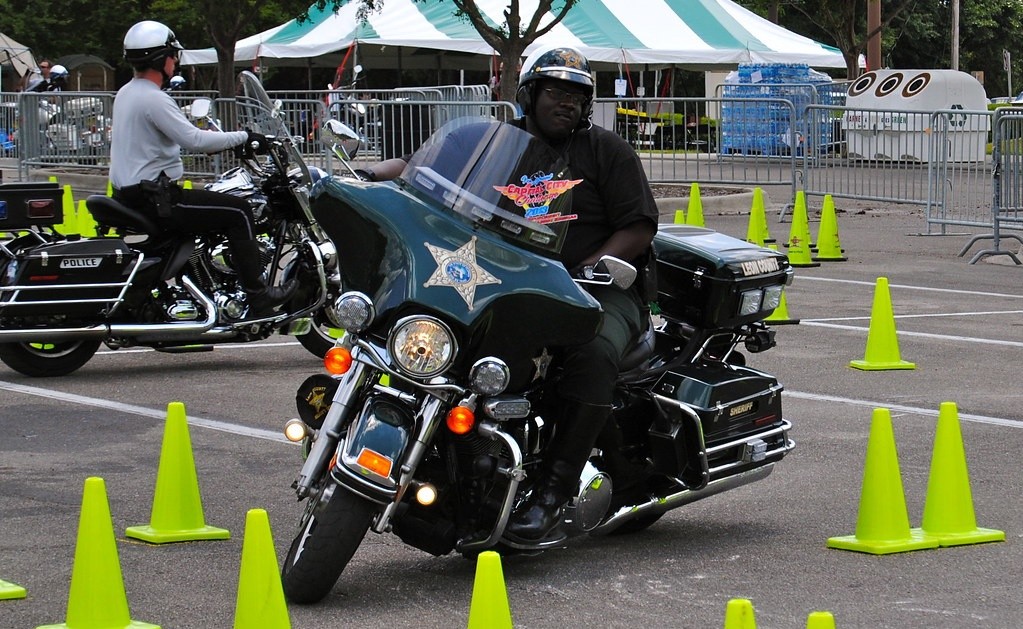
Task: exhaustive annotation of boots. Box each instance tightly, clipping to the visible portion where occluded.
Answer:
[501,398,616,544]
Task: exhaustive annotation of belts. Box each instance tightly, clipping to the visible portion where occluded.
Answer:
[110,182,179,200]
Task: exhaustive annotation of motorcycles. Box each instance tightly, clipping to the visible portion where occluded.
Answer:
[1,64,348,377]
[284,114,798,604]
[316,65,373,151]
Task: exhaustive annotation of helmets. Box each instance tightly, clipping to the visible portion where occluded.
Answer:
[120,21,185,62]
[169,75,187,87]
[49,64,70,79]
[516,45,595,97]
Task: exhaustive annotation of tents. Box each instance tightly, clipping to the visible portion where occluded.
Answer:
[177,0,847,113]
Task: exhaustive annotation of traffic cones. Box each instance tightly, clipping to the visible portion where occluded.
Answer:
[761,290,801,325]
[850,277,917,371]
[911,401,1006,547]
[125,402,229,545]
[722,597,839,629]
[673,182,848,268]
[234,508,292,629]
[465,551,512,629]
[827,407,940,557]
[35,477,162,629]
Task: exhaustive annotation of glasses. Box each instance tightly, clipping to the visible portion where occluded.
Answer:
[542,86,587,105]
[40,66,49,68]
[167,52,178,59]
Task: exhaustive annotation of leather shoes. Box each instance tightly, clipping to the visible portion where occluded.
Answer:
[254,278,300,320]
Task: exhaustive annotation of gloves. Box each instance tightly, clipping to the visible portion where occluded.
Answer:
[245,131,267,152]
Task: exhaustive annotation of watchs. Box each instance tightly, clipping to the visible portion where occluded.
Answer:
[573,261,596,281]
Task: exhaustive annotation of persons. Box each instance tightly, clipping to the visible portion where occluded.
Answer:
[109,20,301,317]
[350,47,661,547]
[493,62,522,122]
[16,60,64,150]
